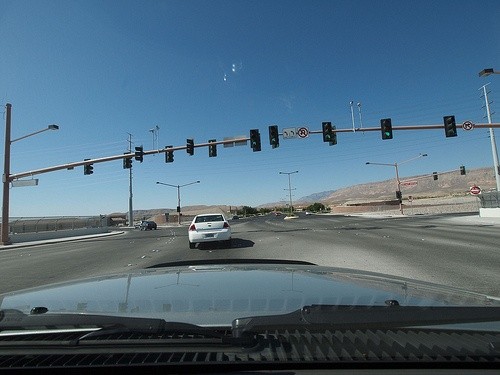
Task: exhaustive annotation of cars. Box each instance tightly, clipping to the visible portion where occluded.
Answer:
[188,214,232,249]
[139,221,157,232]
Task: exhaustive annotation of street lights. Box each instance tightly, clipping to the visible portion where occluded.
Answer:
[156,181,201,225]
[280,171,299,212]
[1,103,59,246]
[365,162,403,215]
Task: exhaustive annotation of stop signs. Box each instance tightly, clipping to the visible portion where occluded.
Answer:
[470,186,481,195]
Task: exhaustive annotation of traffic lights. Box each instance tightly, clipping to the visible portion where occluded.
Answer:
[380,118,393,140]
[166,146,175,162]
[83,159,94,175]
[322,122,333,142]
[268,125,279,145]
[443,116,457,138]
[186,139,194,155]
[123,153,132,169]
[250,129,260,148]
